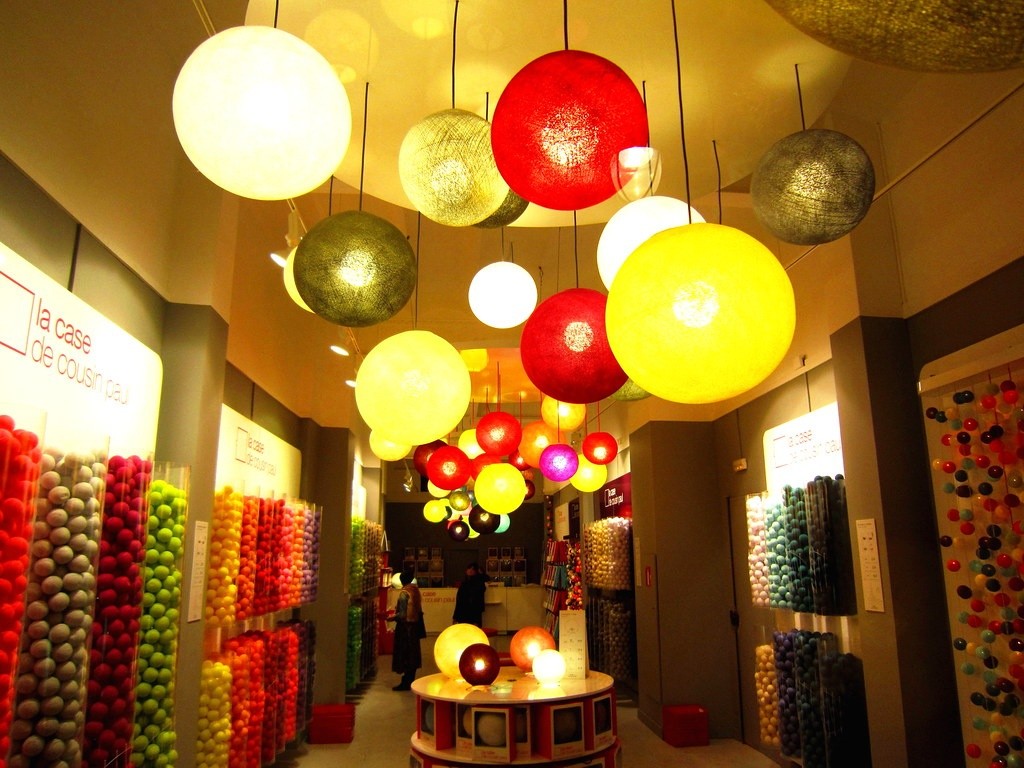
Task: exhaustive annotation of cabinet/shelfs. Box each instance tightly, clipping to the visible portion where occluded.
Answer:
[409,669,623,768]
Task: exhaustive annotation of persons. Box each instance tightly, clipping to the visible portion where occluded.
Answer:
[452,564,487,629]
[386,571,427,692]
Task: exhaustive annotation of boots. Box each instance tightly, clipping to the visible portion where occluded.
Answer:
[392,675,412,691]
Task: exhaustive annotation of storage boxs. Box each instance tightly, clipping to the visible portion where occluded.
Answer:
[745,474,873,768]
[0,395,386,768]
[404,548,444,588]
[486,547,527,587]
[583,519,638,699]
[662,702,710,747]
[415,692,614,764]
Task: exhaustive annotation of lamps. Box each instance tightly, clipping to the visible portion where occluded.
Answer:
[170,0,1024,539]
[433,622,567,690]
[383,567,392,587]
